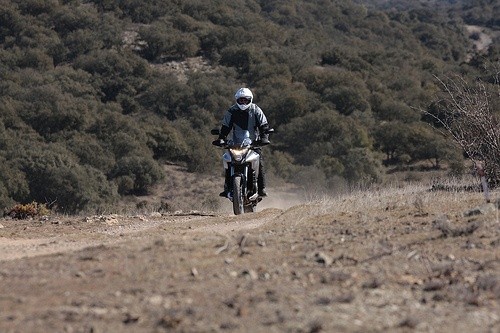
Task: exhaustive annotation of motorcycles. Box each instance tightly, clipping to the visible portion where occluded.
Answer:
[211,128,275,215]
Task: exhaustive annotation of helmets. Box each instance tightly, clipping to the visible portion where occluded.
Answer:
[235,88,253,110]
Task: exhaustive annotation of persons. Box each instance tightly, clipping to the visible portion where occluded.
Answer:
[214,88,271,198]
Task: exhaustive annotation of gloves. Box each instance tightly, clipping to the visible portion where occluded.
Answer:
[212,140,223,147]
[261,138,269,146]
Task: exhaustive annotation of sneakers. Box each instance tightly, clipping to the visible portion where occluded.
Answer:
[219,190,228,198]
[258,187,266,196]
